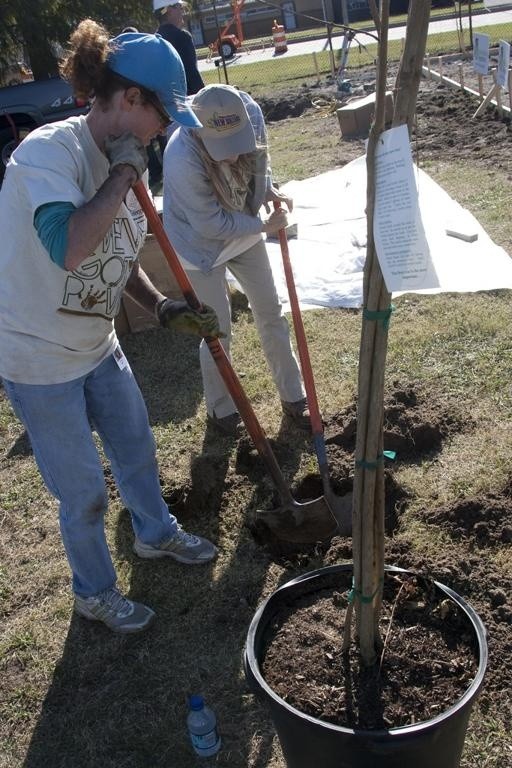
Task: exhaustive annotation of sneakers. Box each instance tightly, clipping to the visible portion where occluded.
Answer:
[133,524,219,564]
[72,585,157,635]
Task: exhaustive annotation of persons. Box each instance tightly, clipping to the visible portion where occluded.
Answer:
[500,45,505,73]
[120,25,142,33]
[154,0,206,95]
[164,80,324,439]
[1,17,227,637]
[474,36,481,61]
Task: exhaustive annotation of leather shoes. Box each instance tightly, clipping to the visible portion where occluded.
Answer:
[281,396,325,427]
[207,410,250,434]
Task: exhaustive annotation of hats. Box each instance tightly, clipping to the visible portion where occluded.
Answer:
[190,83,258,161]
[103,33,204,128]
[152,0,188,11]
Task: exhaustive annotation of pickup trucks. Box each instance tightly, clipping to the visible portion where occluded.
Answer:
[0,74,94,181]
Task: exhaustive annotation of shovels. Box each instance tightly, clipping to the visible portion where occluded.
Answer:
[268,173,351,532]
[129,174,343,552]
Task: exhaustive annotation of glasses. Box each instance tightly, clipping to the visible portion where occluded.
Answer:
[129,85,175,130]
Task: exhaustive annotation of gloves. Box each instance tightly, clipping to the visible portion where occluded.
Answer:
[155,297,219,339]
[105,130,149,185]
